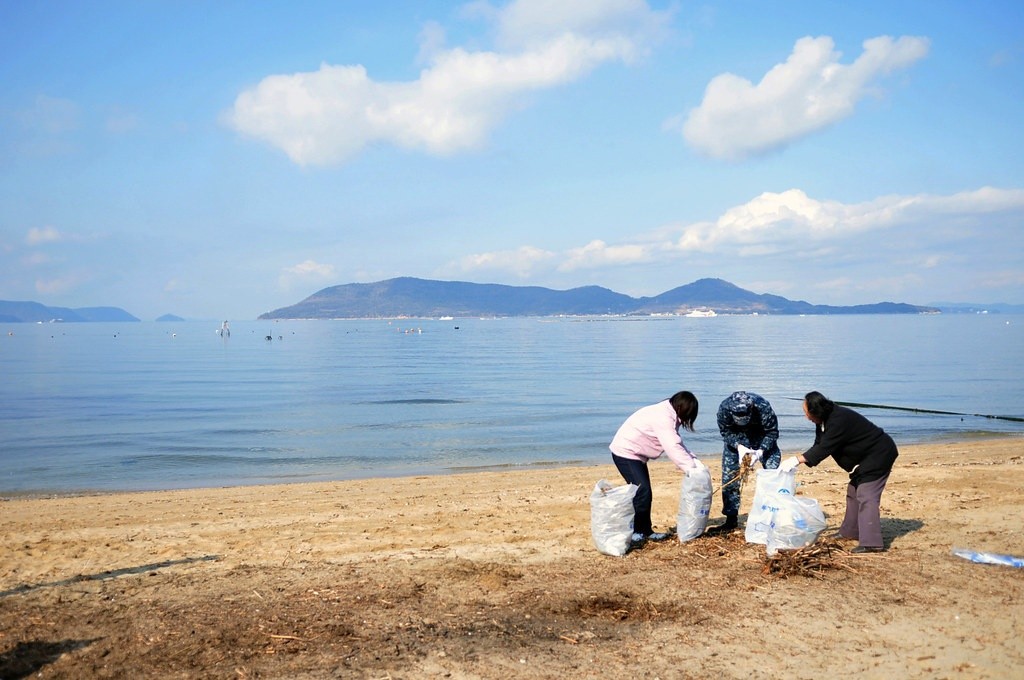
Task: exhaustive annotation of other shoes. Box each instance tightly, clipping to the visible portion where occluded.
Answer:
[718,514,738,530]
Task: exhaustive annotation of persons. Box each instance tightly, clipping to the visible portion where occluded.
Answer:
[609,391,699,542]
[778,391,899,554]
[707,390,782,535]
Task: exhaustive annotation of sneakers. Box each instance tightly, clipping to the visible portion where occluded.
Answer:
[647,533,671,541]
[630,534,656,549]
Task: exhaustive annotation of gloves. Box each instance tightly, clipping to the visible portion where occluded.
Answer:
[778,456,799,472]
[738,444,754,464]
[748,449,763,466]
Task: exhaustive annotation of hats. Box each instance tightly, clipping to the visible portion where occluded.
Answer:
[729,391,753,425]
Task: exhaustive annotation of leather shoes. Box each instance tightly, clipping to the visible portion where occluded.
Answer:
[852,546,888,556]
[827,533,859,542]
